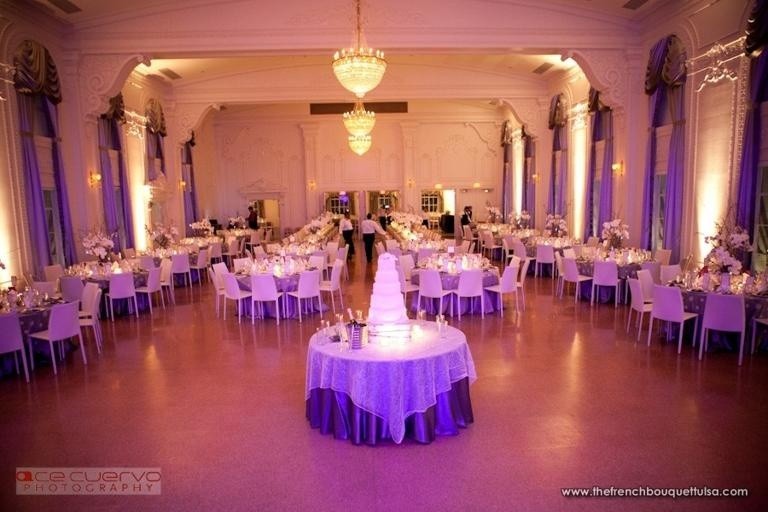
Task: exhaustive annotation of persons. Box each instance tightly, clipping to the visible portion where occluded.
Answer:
[245,206,257,230]
[420,208,429,229]
[460,207,472,236]
[379,205,388,231]
[339,212,355,256]
[465,207,472,220]
[360,213,388,263]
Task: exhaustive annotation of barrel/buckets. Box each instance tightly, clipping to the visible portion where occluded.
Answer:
[346,323,368,348]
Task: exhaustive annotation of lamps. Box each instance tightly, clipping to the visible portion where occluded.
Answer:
[331,0,388,155]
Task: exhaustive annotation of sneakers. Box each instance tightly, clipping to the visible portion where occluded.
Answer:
[346,253,374,263]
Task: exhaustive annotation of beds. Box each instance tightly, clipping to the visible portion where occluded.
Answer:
[303,319,476,446]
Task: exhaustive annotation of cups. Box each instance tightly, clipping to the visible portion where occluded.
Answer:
[0,287,49,313]
[436,314,444,329]
[438,321,447,338]
[417,309,427,324]
[317,308,369,349]
